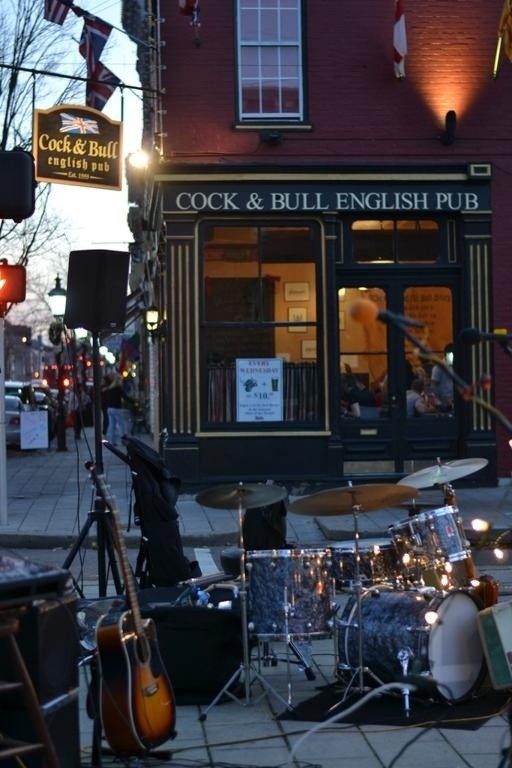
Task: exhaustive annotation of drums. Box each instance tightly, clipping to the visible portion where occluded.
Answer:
[387,504,472,576]
[247,548,335,642]
[326,537,396,587]
[338,584,488,707]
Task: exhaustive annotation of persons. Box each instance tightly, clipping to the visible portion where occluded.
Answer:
[378,342,455,418]
[42,368,149,446]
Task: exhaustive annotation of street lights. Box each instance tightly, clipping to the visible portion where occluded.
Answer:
[47,276,71,452]
[73,325,116,438]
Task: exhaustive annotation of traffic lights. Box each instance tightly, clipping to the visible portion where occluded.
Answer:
[0,265,25,303]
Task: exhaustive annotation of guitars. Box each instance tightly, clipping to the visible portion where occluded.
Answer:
[85,461,177,755]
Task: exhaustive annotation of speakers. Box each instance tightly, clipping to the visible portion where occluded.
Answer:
[63,249,129,334]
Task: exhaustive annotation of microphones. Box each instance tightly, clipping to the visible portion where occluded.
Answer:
[455,326,512,345]
[349,298,424,329]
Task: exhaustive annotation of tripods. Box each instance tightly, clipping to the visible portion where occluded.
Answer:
[61,331,123,598]
[197,491,396,720]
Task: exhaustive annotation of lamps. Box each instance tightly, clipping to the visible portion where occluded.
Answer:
[442,110,456,145]
[259,130,284,144]
[145,302,166,343]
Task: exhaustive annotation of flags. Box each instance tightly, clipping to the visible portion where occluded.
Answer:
[177,1,200,26]
[86,60,121,111]
[79,11,113,65]
[59,112,100,136]
[392,0,408,77]
[498,1,512,63]
[43,0,73,25]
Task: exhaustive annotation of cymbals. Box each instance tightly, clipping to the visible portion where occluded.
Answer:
[287,484,417,515]
[194,483,291,509]
[397,458,489,490]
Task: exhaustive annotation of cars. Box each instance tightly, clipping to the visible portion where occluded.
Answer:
[5,379,56,446]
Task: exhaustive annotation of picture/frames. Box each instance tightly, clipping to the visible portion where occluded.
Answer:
[288,306,307,333]
[300,338,316,360]
[339,311,346,330]
[284,281,310,302]
